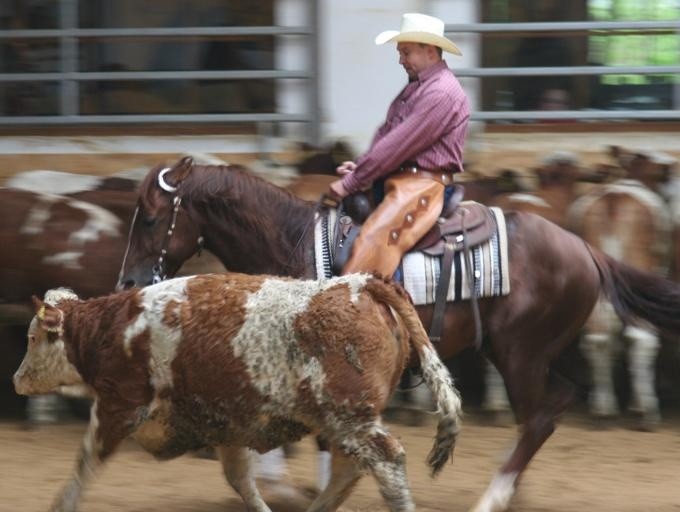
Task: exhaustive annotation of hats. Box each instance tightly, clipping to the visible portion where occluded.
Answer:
[376,12,463,59]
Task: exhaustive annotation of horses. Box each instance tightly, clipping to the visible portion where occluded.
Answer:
[114,156,680,512]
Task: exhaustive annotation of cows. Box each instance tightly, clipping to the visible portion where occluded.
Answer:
[1,138,680,432]
[12,271,468,511]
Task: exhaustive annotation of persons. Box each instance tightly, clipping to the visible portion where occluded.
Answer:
[319,12,470,283]
